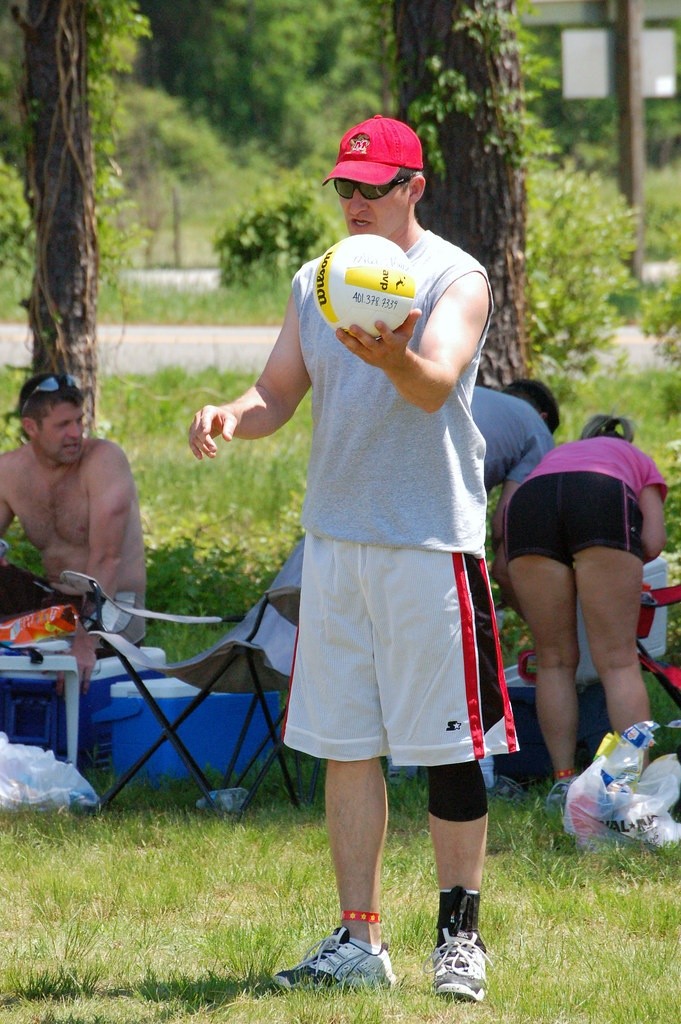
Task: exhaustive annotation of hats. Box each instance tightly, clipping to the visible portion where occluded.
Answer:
[320,114,422,185]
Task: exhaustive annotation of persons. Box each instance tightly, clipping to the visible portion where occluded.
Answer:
[0,374,146,696]
[493,414,669,788]
[386,379,560,790]
[188,113,518,1004]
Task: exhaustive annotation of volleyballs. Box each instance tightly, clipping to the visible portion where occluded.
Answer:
[313,234,416,340]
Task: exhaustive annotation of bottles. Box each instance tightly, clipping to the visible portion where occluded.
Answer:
[196,788,249,810]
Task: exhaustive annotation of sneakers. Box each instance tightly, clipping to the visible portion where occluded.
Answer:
[272,926,396,990]
[423,926,491,1002]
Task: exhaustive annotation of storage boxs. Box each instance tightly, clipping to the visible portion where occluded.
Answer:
[93,677,280,781]
[0,639,78,767]
[0,647,165,772]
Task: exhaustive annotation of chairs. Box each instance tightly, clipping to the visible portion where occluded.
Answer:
[58,537,307,818]
[502,556,681,713]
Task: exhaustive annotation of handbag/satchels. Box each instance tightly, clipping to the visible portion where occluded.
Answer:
[564,754,681,848]
[0,731,101,815]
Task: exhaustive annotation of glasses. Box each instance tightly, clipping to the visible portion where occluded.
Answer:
[333,173,413,200]
[23,374,83,415]
